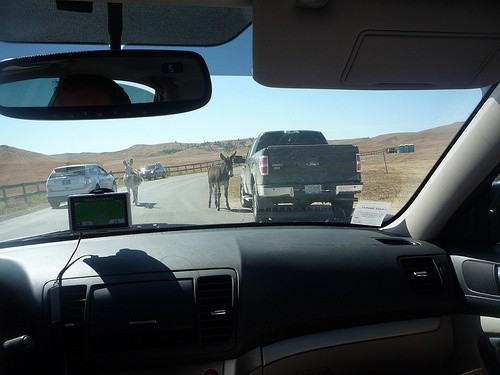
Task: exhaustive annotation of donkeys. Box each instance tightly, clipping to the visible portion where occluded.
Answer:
[207,151,238,211]
[121,158,144,207]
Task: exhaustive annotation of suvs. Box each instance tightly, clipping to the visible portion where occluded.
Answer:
[46,163,118,208]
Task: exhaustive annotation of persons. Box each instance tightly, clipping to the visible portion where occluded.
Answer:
[47,73,131,119]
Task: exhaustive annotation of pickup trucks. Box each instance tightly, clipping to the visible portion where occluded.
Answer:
[239,130,364,224]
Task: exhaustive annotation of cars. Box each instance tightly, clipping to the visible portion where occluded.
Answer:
[140,163,167,180]
[386,148,397,154]
